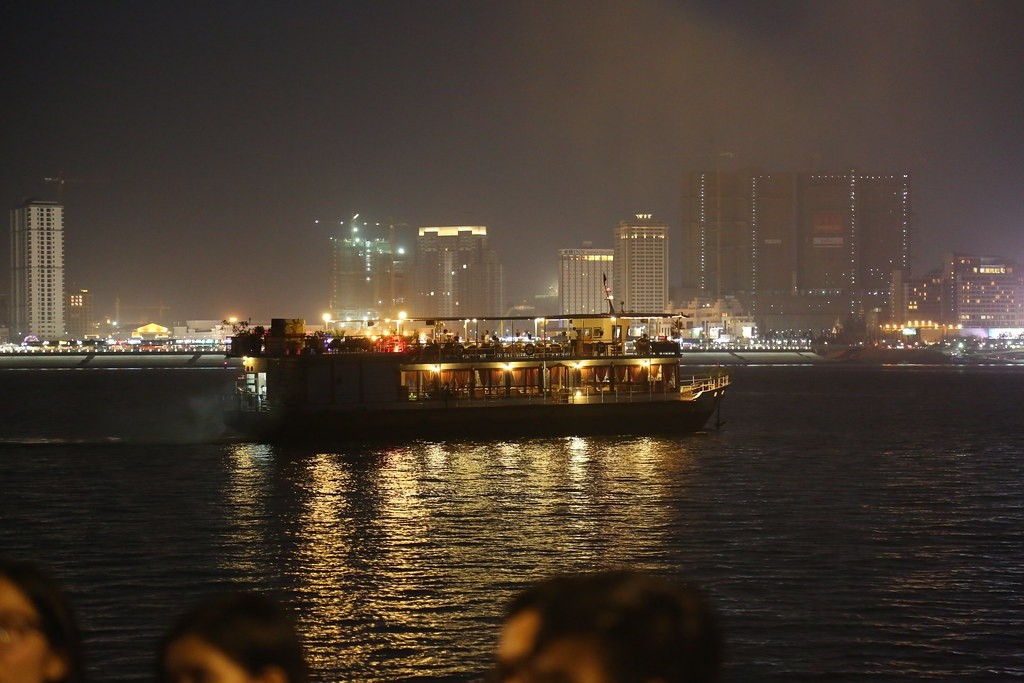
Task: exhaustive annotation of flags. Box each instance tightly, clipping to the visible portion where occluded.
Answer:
[603,272,609,297]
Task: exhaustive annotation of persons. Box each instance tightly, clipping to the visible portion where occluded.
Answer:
[0,561,92,683]
[443,382,450,391]
[154,587,311,683]
[492,566,727,683]
[415,327,653,356]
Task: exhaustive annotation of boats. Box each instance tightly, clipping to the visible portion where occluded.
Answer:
[291,271,733,434]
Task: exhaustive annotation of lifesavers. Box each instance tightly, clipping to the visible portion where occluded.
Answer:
[455,344,464,357]
[596,342,606,353]
[524,344,535,355]
